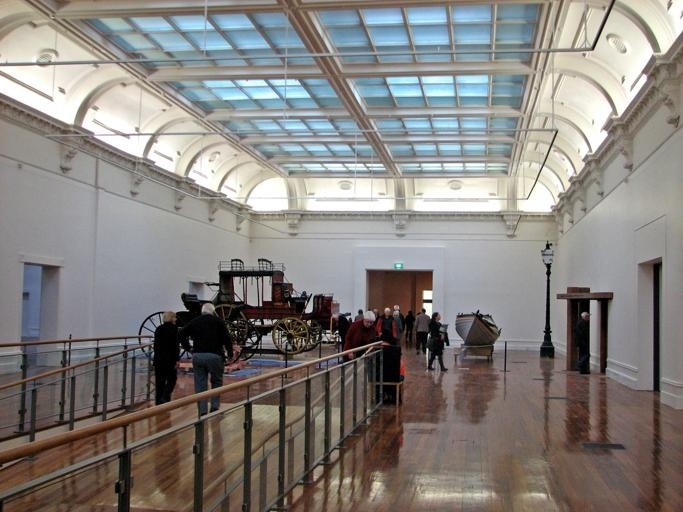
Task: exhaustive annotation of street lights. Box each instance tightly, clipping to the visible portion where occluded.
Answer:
[539,240,555,358]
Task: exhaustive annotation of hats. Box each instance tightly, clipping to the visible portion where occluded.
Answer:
[363,311,375,321]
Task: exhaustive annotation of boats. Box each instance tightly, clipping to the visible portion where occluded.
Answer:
[454,309,503,346]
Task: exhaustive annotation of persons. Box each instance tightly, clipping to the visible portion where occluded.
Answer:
[414,309,430,354]
[152,409,227,503]
[179,303,234,416]
[354,309,363,321]
[369,305,404,344]
[425,312,449,371]
[573,312,589,374]
[337,312,352,353]
[565,374,589,449]
[403,310,415,345]
[377,332,406,405]
[153,310,180,405]
[425,371,448,424]
[343,310,375,364]
[378,420,404,472]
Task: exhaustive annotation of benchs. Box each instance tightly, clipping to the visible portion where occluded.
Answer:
[181,293,212,311]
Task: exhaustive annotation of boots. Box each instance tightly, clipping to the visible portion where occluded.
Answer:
[440,360,448,371]
[428,359,434,370]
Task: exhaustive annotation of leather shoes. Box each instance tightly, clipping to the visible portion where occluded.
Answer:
[209,407,218,412]
